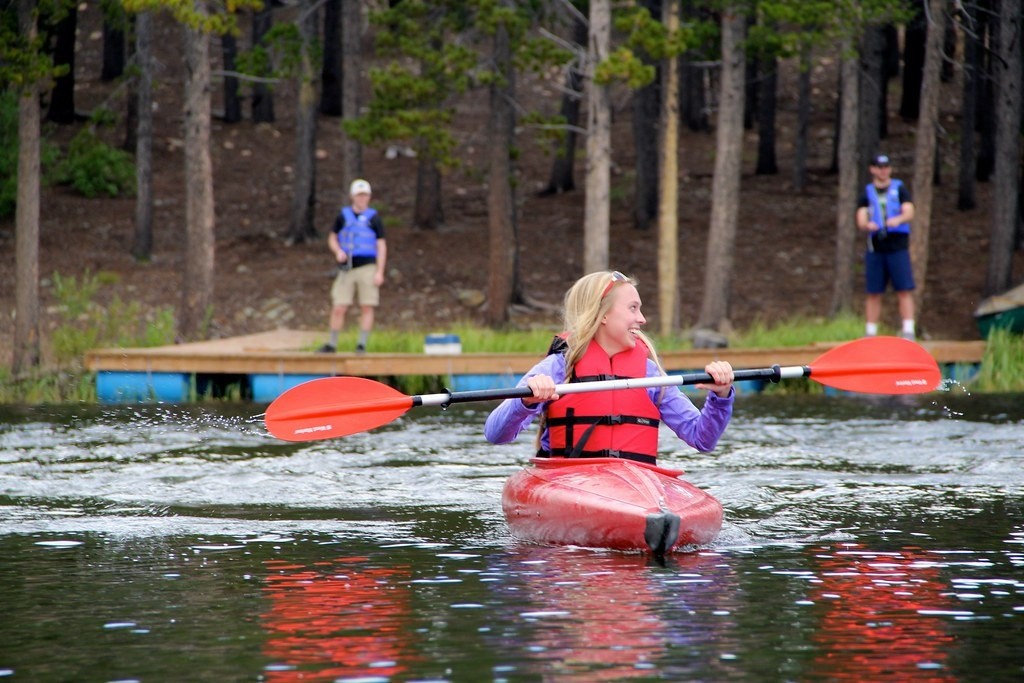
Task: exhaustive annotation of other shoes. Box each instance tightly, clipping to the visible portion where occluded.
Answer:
[317,343,366,355]
[902,332,916,343]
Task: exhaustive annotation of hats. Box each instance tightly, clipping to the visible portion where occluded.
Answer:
[349,178,372,196]
[870,153,891,166]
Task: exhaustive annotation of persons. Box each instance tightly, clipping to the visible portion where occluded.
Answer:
[482,266,737,473]
[855,152,919,343]
[314,180,387,355]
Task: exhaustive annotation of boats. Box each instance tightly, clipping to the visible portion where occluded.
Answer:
[500,456,724,552]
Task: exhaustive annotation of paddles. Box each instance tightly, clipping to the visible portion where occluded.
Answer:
[263,333,943,444]
